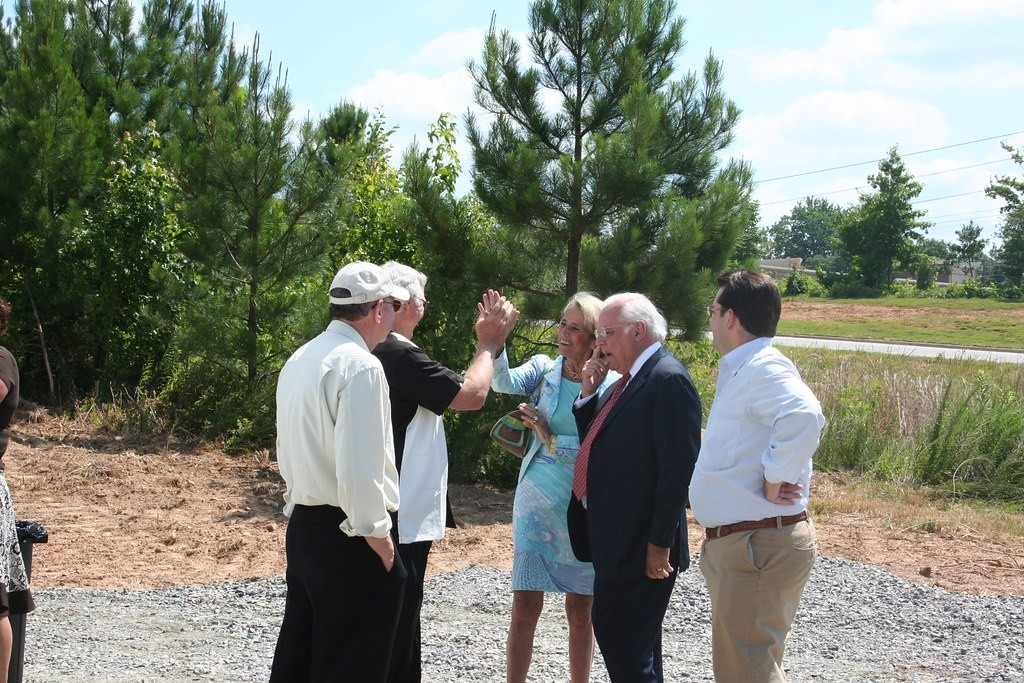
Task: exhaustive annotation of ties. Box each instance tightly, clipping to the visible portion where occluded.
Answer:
[573,372,631,502]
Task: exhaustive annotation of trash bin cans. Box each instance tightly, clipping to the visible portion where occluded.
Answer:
[6,521,46,683]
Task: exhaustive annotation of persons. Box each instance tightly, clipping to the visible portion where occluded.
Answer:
[477,289,622,683]
[689,270,825,683]
[371,261,516,683]
[567,292,702,683]
[270,261,410,683]
[0,298,35,683]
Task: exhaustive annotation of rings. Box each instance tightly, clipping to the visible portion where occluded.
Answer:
[657,568,662,571]
[532,406,540,425]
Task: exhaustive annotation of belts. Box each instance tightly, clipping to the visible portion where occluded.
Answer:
[706,511,807,539]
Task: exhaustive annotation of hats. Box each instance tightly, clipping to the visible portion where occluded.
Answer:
[329,260,411,305]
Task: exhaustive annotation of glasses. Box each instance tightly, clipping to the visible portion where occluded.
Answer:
[409,295,431,311]
[707,306,729,317]
[594,320,637,340]
[371,300,402,312]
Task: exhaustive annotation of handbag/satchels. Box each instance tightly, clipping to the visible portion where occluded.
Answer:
[489,360,555,458]
